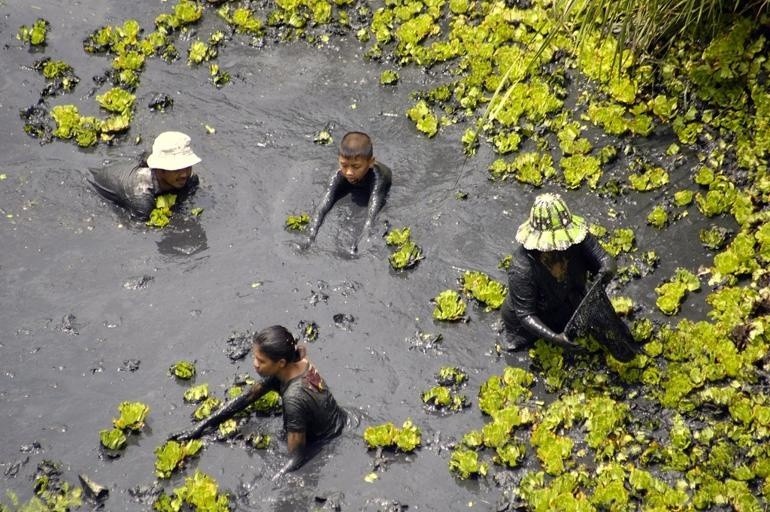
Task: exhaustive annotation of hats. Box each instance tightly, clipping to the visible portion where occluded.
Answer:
[147,131,202,171]
[515,192,589,254]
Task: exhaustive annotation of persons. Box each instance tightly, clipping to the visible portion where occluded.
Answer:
[89,131,202,222]
[298,133,392,255]
[500,192,617,352]
[167,325,347,482]
